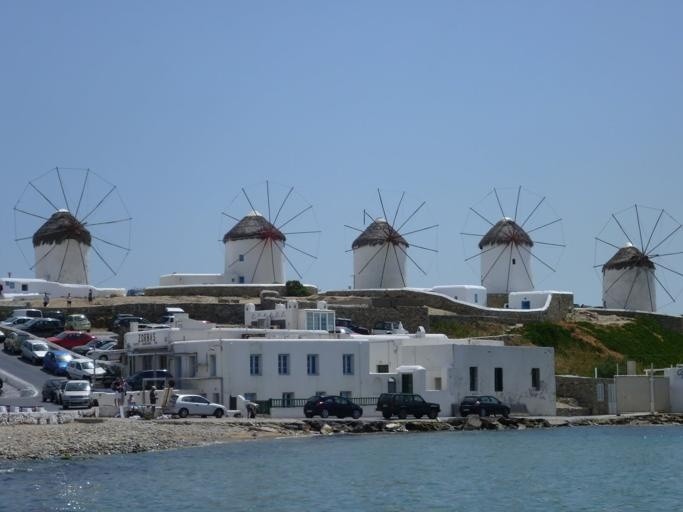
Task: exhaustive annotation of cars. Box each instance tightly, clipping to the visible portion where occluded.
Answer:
[0,308,211,410]
[304,395,363,419]
[230,395,257,418]
[333,317,410,337]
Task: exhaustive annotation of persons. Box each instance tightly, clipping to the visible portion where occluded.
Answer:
[127,394,138,412]
[150,385,160,414]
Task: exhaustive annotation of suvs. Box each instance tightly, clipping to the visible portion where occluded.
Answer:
[459,394,510,418]
[375,391,440,419]
[166,393,226,420]
